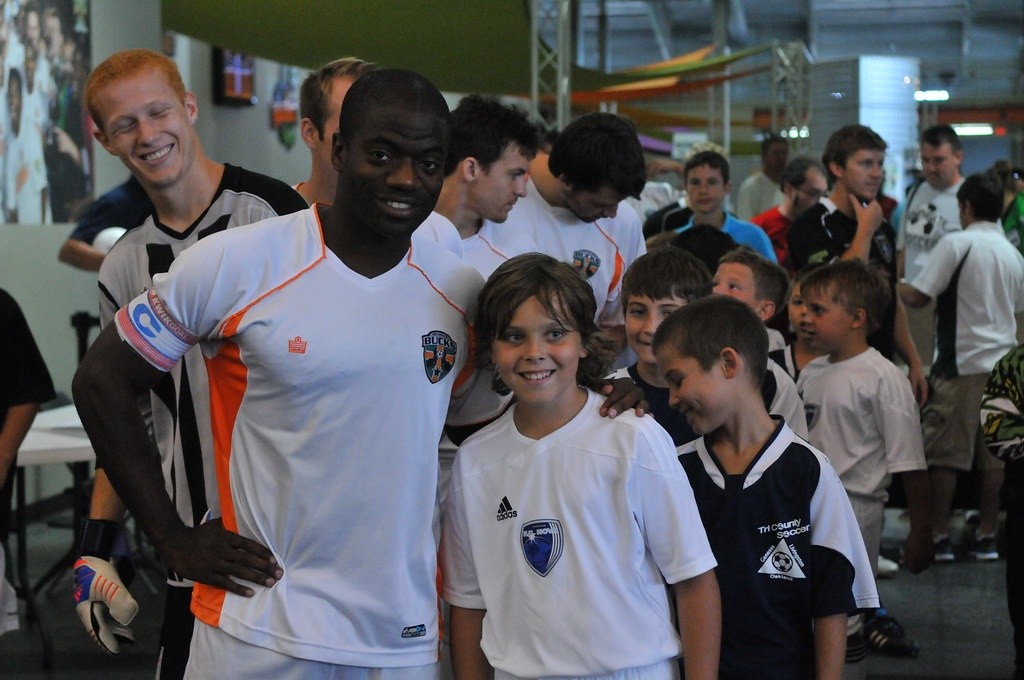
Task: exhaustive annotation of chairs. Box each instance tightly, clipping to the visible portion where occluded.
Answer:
[43,311,135,595]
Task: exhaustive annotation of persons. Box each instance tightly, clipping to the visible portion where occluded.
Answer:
[56,51,1024,680]
[651,293,880,680]
[0,0,96,224]
[444,252,722,680]
[0,289,57,633]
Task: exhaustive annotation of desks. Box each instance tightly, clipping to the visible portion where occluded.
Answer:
[11,403,135,680]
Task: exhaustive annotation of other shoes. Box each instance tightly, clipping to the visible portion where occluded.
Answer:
[879,556,899,576]
[966,527,998,559]
[842,661,867,680]
[876,616,905,638]
[934,534,953,560]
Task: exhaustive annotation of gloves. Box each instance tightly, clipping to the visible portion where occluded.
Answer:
[72,520,139,657]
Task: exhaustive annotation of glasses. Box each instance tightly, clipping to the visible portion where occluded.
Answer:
[799,188,828,201]
